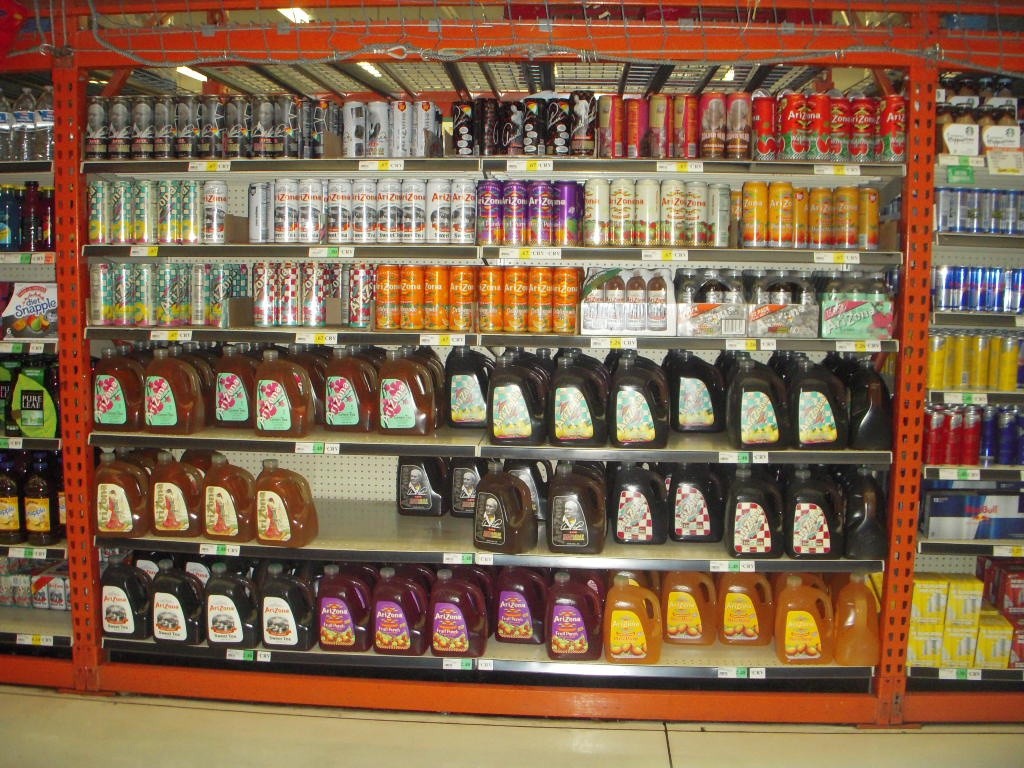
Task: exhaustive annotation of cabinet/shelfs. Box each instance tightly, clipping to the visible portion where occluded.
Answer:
[0,0,1024,728]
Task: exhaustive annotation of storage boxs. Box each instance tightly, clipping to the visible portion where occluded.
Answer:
[909,555,1024,670]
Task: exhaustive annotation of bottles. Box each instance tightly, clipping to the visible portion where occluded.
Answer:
[92,340,894,449]
[821,271,889,294]
[0,84,55,162]
[583,268,668,331]
[0,351,60,439]
[99,543,883,668]
[937,75,1017,154]
[0,449,67,546]
[749,270,816,304]
[0,179,55,253]
[88,437,890,563]
[678,270,744,304]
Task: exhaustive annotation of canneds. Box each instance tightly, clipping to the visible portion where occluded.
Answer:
[881,186,1024,236]
[375,263,585,334]
[752,94,905,164]
[922,400,1024,466]
[248,177,731,248]
[252,262,379,329]
[86,94,343,159]
[884,263,1024,315]
[87,179,228,245]
[342,100,443,159]
[731,181,881,251]
[883,327,1024,392]
[452,89,751,160]
[89,262,248,329]
